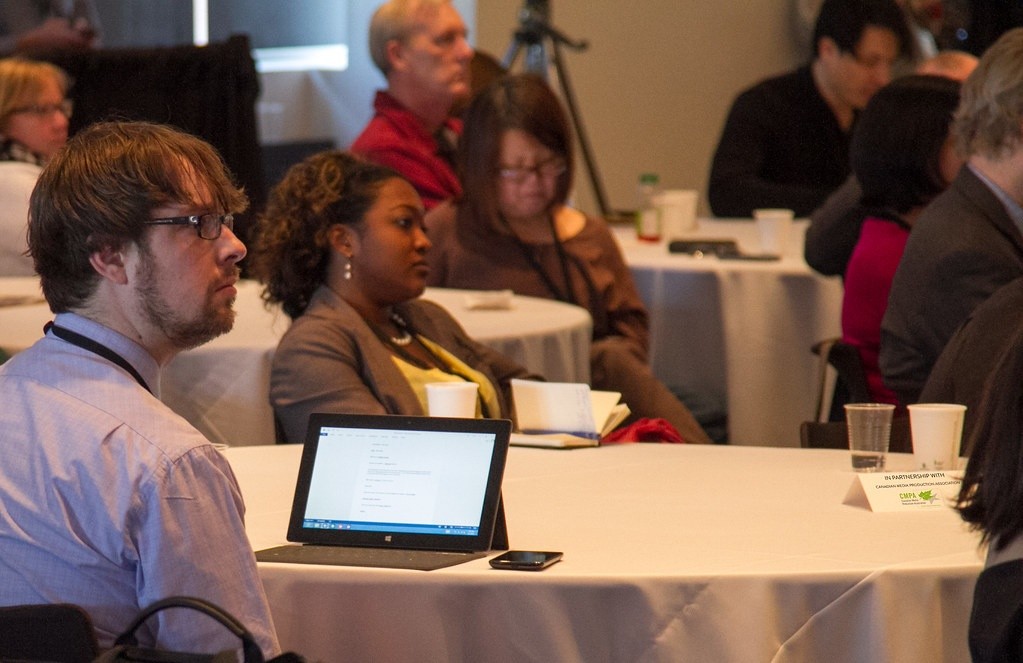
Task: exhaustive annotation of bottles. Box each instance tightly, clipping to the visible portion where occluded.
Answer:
[634,174,662,242]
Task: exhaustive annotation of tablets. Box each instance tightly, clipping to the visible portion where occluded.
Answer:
[287,413,513,555]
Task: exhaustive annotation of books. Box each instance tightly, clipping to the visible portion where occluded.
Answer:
[510,378,630,448]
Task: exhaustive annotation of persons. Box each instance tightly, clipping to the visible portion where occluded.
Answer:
[0,58,71,279]
[423,74,714,446]
[0,0,105,57]
[1,122,283,663]
[880,26,1023,663]
[805,51,977,275]
[708,0,914,220]
[346,0,474,214]
[840,76,962,420]
[252,150,692,443]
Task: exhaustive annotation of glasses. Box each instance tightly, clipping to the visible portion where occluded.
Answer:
[498,157,567,180]
[12,98,72,115]
[848,48,908,72]
[138,214,233,240]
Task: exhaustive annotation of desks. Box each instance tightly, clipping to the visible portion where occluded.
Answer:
[0,285,598,449]
[226,438,996,663]
[601,214,843,448]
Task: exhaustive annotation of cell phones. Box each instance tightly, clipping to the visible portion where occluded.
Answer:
[489,550,564,571]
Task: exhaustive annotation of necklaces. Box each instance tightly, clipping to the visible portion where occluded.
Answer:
[390,314,411,345]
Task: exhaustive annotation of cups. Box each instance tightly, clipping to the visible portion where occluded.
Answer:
[908,403,967,472]
[753,208,793,256]
[844,403,895,472]
[657,188,699,238]
[424,380,480,418]
